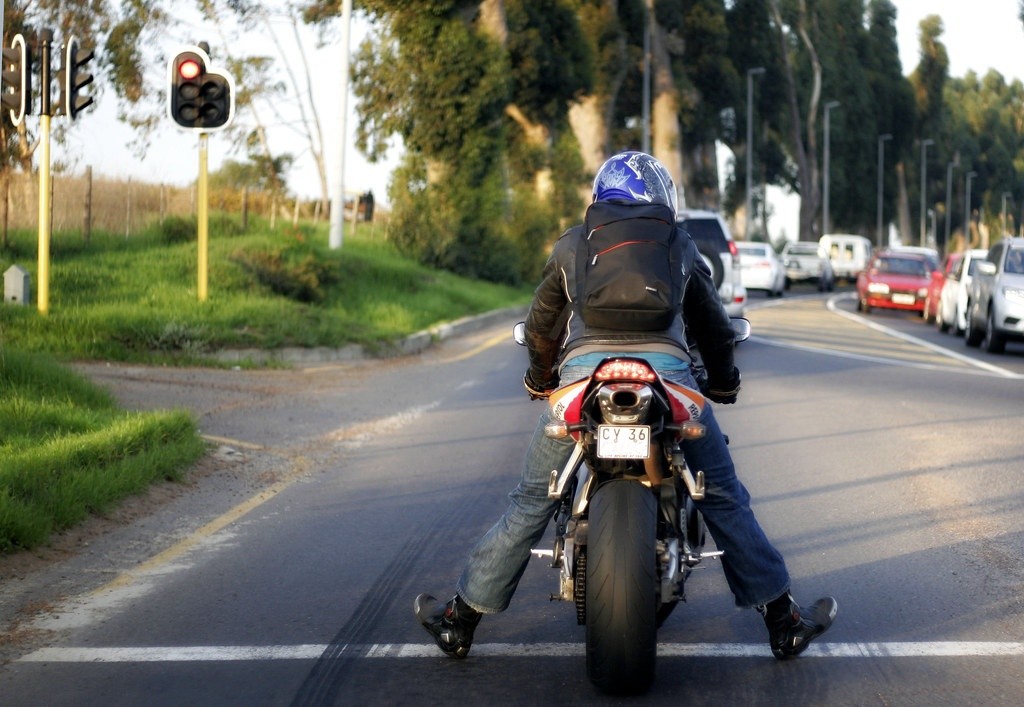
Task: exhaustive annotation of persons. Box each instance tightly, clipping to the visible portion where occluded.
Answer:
[413,150,839,658]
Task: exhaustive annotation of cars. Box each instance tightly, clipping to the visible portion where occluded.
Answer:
[924,247,993,335]
[855,245,941,314]
[734,242,787,298]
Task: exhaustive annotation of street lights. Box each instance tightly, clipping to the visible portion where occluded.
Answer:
[945,162,1011,247]
[822,100,841,234]
[919,138,935,244]
[745,66,769,242]
[876,132,894,243]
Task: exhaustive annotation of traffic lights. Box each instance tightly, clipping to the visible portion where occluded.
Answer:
[2,33,27,126]
[167,45,236,132]
[65,35,93,122]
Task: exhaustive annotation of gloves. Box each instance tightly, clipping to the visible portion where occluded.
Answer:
[523,367,556,397]
[707,366,741,405]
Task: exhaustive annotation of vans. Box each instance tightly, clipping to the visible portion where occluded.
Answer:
[819,234,872,283]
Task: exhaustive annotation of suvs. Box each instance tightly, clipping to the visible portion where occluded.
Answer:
[675,209,750,323]
[965,237,1024,355]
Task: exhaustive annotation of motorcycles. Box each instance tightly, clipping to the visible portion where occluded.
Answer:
[514,310,752,700]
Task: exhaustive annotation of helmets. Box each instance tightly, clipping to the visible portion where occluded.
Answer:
[592,151,679,222]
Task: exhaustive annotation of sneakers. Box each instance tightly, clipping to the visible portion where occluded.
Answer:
[415,593,473,661]
[771,597,838,659]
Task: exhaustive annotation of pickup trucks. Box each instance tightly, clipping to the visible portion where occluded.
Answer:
[780,243,836,292]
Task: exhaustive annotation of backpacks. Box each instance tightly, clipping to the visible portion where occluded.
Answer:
[575,202,681,332]
